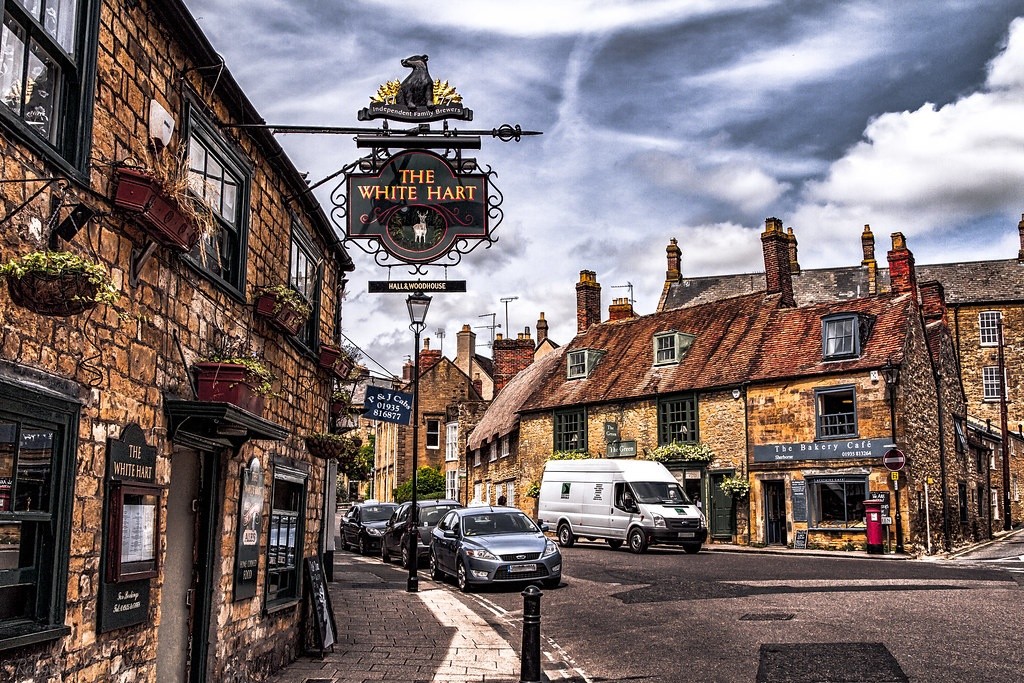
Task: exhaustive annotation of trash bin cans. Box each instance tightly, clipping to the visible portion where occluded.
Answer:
[305,556,338,647]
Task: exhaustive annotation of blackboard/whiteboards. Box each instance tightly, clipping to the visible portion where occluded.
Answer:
[789,480,809,522]
[797,529,808,547]
[869,490,890,518]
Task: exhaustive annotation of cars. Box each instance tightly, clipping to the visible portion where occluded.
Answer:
[379,497,466,569]
[428,504,563,594]
[340,502,400,556]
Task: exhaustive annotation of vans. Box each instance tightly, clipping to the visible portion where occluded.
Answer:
[537,458,708,555]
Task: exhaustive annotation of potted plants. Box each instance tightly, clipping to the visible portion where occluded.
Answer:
[306,432,348,458]
[331,390,351,418]
[256,284,309,335]
[0,249,118,317]
[192,357,273,417]
[319,345,362,379]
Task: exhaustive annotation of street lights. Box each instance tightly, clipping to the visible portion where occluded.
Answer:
[405,289,437,594]
[877,354,906,554]
